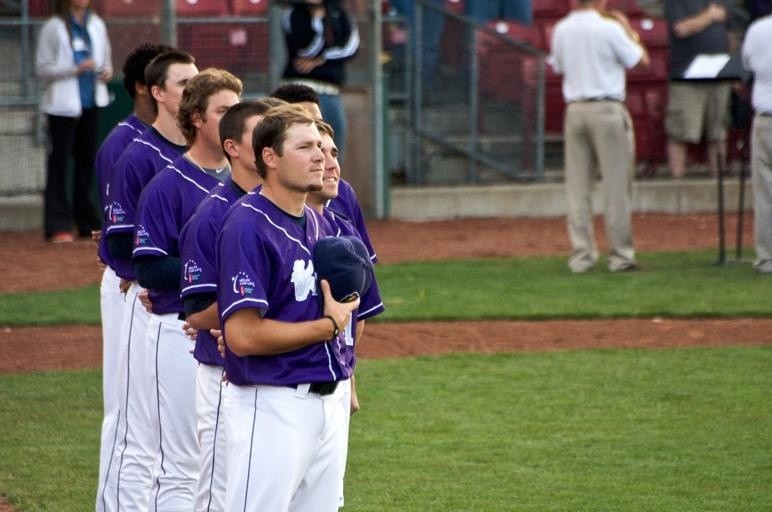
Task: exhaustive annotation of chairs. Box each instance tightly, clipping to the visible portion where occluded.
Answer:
[98,0,268,81]
[438,1,752,167]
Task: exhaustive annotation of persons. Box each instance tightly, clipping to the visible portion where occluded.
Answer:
[663,0,740,178]
[32,0,114,243]
[739,13,772,275]
[460,0,534,102]
[546,0,652,271]
[388,0,445,107]
[280,0,361,168]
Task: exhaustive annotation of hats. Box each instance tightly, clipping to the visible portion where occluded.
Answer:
[315,235,375,303]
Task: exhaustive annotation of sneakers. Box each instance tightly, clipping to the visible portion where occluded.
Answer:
[53,232,73,244]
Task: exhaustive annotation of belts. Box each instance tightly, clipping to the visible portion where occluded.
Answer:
[290,380,341,395]
